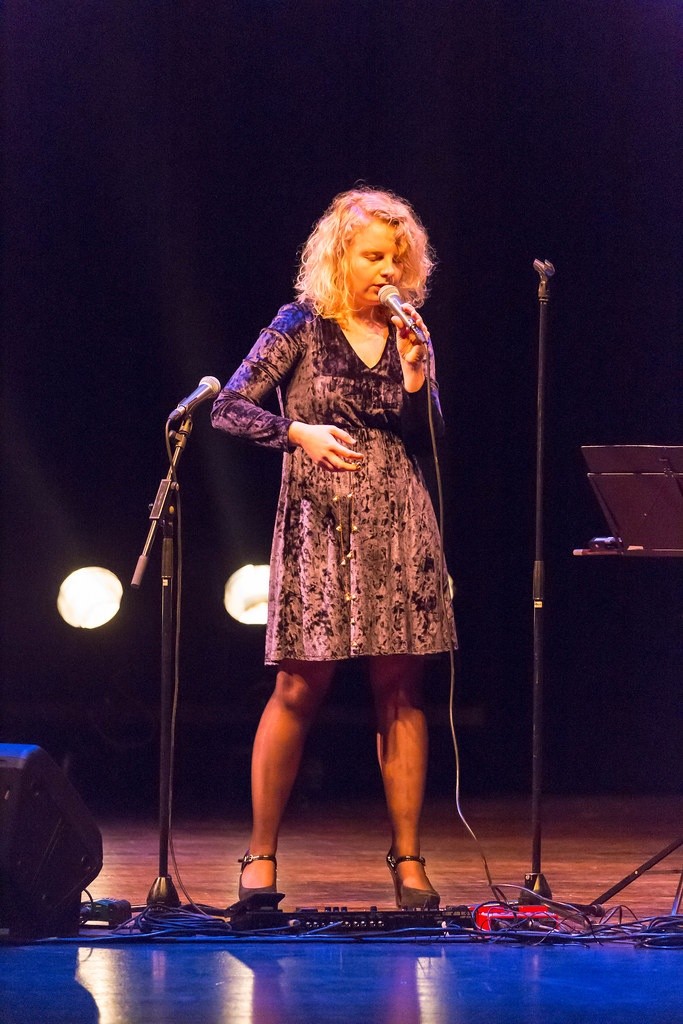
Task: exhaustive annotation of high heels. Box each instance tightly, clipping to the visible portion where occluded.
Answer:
[386,849,441,909]
[237,850,278,900]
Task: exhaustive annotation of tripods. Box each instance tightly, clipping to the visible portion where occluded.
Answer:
[132,412,230,920]
[478,257,604,915]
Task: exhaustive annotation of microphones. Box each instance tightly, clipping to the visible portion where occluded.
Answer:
[378,285,426,346]
[167,376,220,421]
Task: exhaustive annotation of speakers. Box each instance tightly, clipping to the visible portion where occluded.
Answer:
[0,742,103,939]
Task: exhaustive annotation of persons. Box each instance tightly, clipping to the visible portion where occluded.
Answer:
[210,189,459,908]
